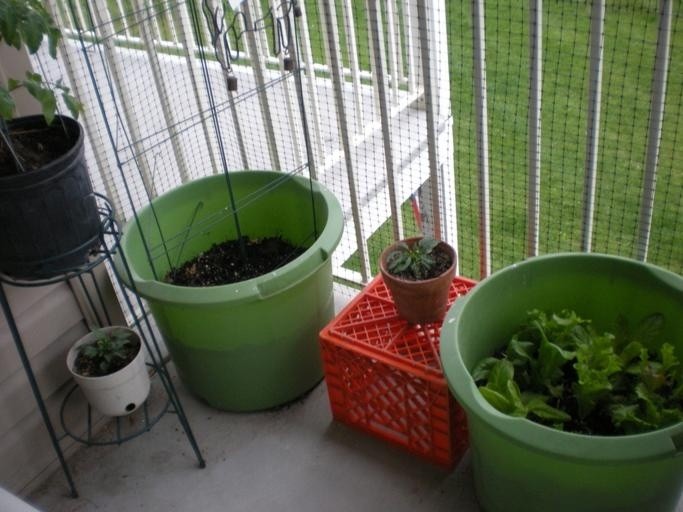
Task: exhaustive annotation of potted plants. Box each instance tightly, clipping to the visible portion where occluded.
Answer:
[66,326,151,417]
[0,0,104,279]
[380,236,456,323]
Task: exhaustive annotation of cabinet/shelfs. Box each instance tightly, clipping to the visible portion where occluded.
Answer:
[0,191,205,498]
